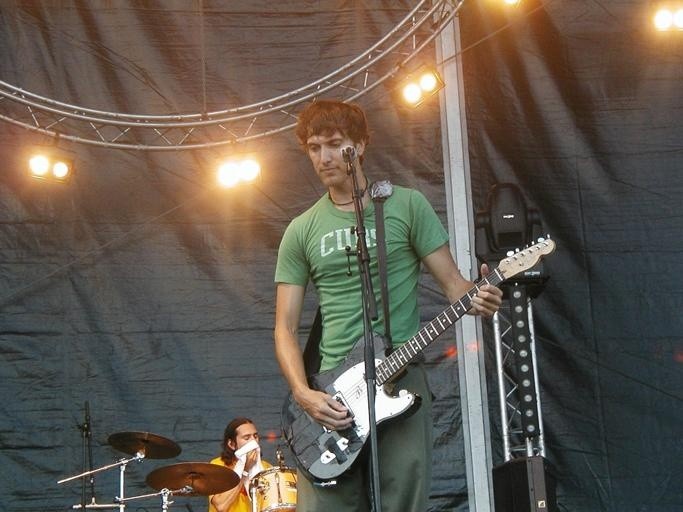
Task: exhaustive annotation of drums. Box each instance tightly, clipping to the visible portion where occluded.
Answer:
[249,466,298,512]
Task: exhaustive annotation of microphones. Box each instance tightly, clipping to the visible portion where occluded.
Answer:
[341,146,357,162]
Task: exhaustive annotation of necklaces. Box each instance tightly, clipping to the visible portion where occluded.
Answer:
[328,173,369,206]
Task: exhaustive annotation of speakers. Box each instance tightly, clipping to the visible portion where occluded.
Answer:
[492,456,557,512]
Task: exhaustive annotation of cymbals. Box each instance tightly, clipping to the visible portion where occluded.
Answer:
[108,430,181,459]
[146,462,241,497]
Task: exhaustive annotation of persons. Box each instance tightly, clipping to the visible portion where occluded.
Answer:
[207,416,273,512]
[273,101,503,512]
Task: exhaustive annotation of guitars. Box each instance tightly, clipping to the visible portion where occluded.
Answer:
[281,232,557,490]
